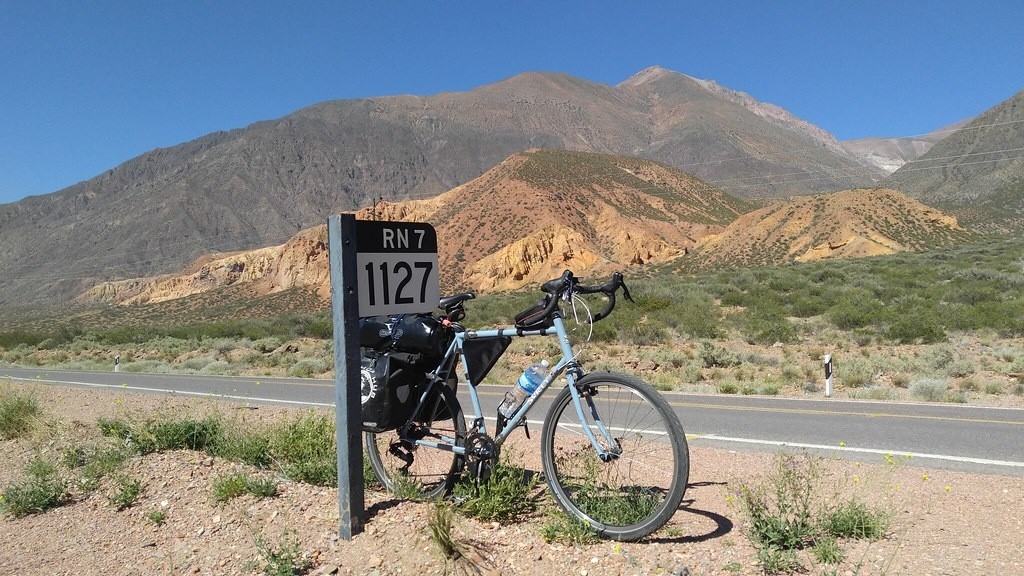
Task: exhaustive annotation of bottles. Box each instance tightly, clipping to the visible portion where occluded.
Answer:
[498,360,549,417]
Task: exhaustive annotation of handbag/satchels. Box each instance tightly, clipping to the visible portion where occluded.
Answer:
[359,314,439,351]
[361,349,420,433]
[418,350,459,421]
[515,295,554,330]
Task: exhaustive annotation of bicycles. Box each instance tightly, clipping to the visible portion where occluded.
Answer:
[361,269,690,542]
